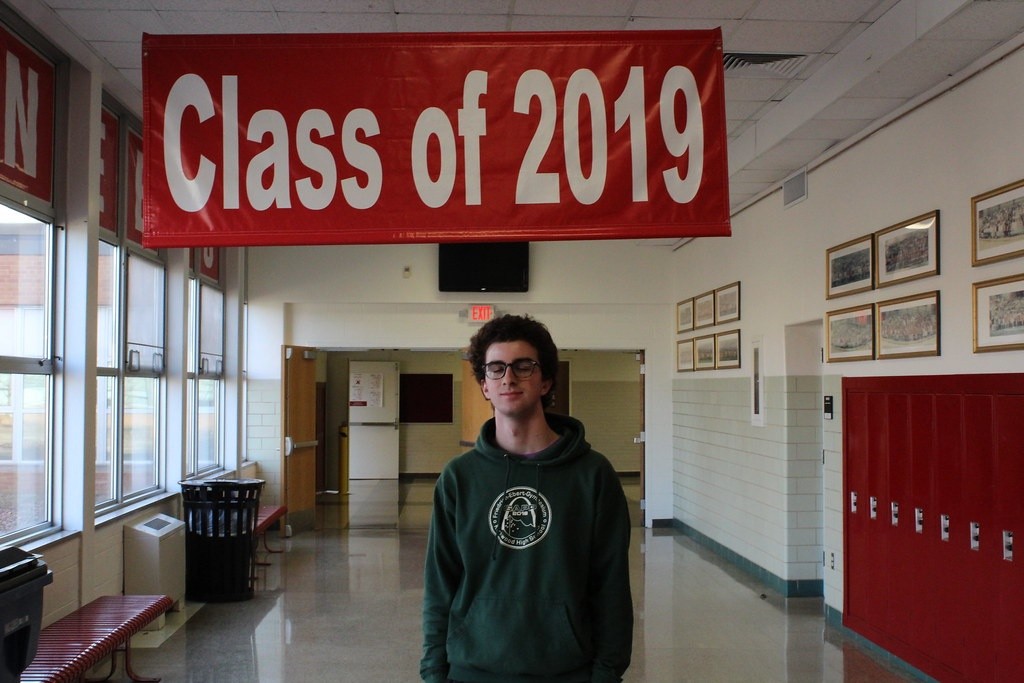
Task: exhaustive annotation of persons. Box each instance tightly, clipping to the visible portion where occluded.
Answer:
[979,200,1024,239]
[882,311,936,341]
[886,234,928,263]
[419,313,633,683]
[991,298,1024,329]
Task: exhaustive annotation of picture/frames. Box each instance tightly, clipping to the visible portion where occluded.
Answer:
[825,303,878,363]
[693,290,715,330]
[694,334,715,371]
[716,329,742,369]
[971,273,1024,353]
[971,179,1024,267]
[875,290,942,360]
[677,338,694,372]
[826,233,875,300]
[714,281,740,326]
[676,297,694,333]
[875,210,940,289]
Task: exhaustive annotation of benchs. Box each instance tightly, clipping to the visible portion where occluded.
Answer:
[244,504,288,588]
[20,595,173,683]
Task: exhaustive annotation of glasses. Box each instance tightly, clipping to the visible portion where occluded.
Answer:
[482,359,542,380]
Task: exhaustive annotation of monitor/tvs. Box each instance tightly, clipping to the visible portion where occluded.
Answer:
[438,240,530,293]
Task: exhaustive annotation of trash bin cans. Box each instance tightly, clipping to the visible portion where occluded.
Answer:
[178,478,266,605]
[0,545,54,683]
